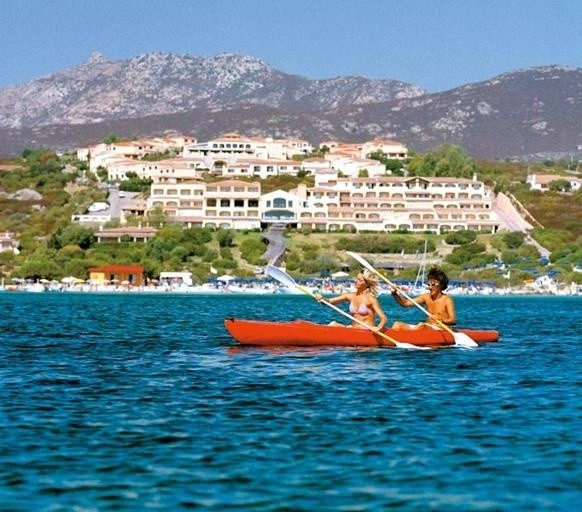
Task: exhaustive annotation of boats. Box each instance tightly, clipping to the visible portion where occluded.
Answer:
[0,240,582,297]
[225,318,500,346]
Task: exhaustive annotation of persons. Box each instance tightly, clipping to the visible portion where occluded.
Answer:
[391,266,457,332]
[314,268,387,334]
[442,277,582,296]
[2,272,357,297]
[378,282,430,295]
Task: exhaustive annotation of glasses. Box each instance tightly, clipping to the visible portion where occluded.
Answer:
[427,283,439,287]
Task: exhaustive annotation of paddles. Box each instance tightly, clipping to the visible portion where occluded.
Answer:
[347,251,478,348]
[267,265,432,351]
